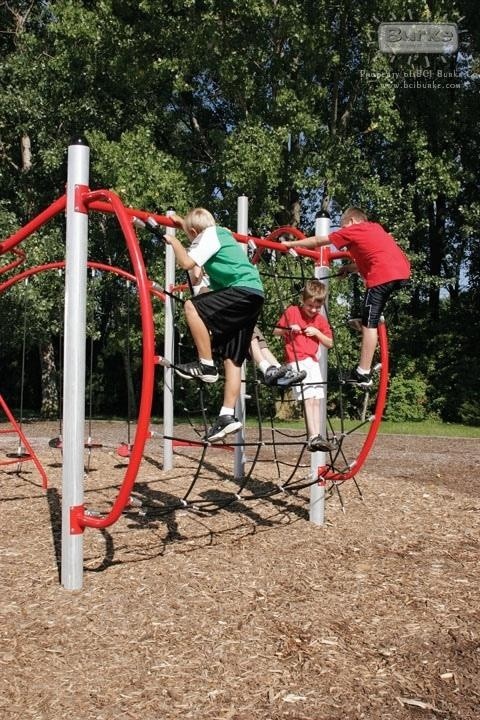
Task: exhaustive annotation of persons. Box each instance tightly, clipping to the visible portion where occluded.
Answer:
[163,207,265,444]
[188,265,307,389]
[272,280,336,451]
[281,207,410,387]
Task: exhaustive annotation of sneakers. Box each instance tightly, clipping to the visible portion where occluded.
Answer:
[264,365,287,387]
[207,413,242,442]
[277,369,307,385]
[309,439,337,453]
[339,350,382,386]
[175,361,220,383]
